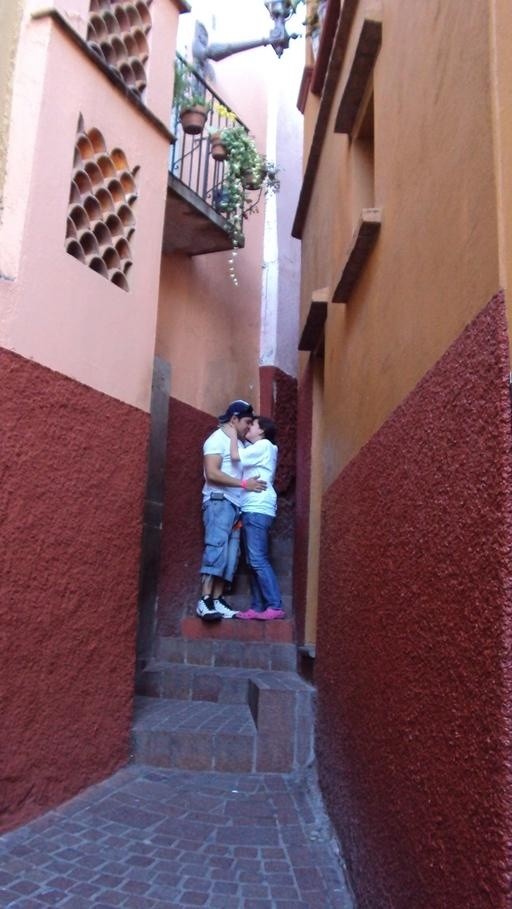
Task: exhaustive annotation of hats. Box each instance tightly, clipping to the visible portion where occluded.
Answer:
[218,400,256,423]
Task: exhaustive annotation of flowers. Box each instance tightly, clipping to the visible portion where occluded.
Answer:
[213,101,236,133]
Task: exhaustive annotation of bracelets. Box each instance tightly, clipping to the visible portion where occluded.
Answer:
[241,478,247,489]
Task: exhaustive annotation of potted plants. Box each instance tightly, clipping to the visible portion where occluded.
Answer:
[213,126,279,251]
[171,58,212,135]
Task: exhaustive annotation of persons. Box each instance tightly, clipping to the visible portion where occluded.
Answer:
[196,399,268,623]
[215,414,287,621]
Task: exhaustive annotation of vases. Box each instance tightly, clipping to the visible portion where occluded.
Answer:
[209,133,227,160]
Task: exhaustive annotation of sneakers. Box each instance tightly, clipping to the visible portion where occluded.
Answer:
[234,608,257,619]
[255,607,287,620]
[213,593,240,619]
[196,594,225,621]
